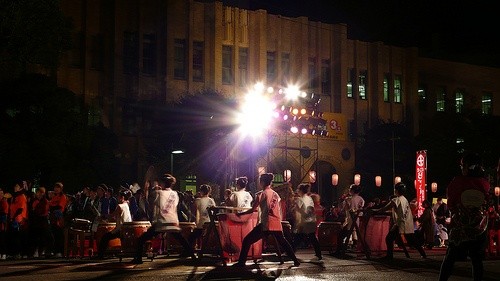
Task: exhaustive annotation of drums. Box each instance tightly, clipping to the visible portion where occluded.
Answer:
[95,222,122,250]
[315,222,344,251]
[120,220,153,257]
[71,218,92,232]
[172,221,196,250]
[279,219,293,239]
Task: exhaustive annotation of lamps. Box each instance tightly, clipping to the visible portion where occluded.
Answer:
[494,186,500,196]
[376,176,381,186]
[309,171,316,183]
[395,176,401,184]
[431,182,437,192]
[354,174,360,185]
[283,169,292,182]
[332,174,338,185]
[264,84,330,138]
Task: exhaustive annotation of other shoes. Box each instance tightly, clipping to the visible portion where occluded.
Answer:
[336,250,345,255]
[317,256,322,260]
[381,256,393,261]
[0,251,62,259]
[90,255,104,260]
[294,261,299,267]
[132,258,142,264]
[232,263,241,268]
[420,255,426,259]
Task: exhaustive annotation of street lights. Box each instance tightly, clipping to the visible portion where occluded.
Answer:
[169,149,185,177]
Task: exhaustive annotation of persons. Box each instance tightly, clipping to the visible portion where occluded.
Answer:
[180,184,217,257]
[232,173,300,268]
[374,181,427,259]
[368,196,500,256]
[221,177,240,214]
[130,174,194,263]
[291,183,323,261]
[437,153,490,281]
[0,175,163,259]
[176,189,196,221]
[308,184,366,257]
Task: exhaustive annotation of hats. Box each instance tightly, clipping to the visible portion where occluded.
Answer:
[98,184,108,192]
[56,182,64,189]
